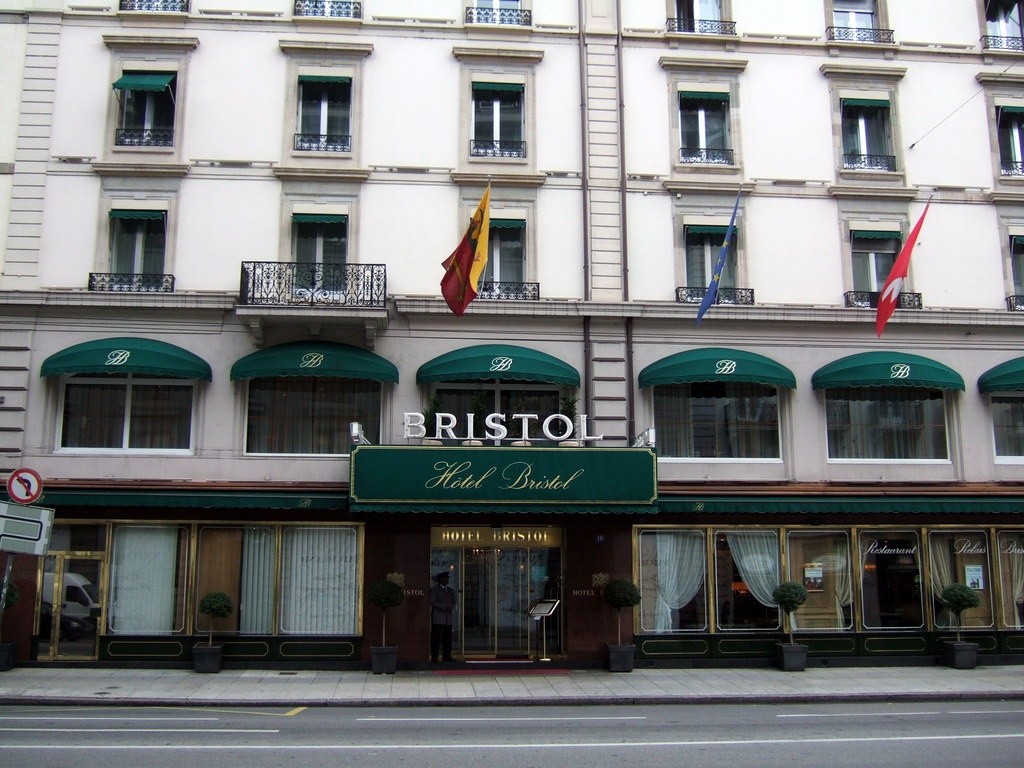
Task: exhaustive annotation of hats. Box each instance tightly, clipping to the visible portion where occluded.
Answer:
[434,571,451,582]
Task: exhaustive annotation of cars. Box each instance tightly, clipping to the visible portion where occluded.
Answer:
[39,604,83,640]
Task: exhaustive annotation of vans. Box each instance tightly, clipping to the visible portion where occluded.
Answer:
[43,573,100,621]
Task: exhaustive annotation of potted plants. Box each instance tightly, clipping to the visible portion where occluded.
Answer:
[601,580,642,672]
[0,581,21,672]
[772,582,809,671]
[192,591,233,673]
[941,583,980,668]
[365,578,403,674]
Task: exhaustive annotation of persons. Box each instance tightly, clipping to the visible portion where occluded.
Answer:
[430,572,455,663]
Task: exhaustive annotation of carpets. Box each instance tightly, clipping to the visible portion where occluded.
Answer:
[432,669,574,675]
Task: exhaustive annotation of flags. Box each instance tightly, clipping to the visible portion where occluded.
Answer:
[876,202,929,340]
[440,185,491,317]
[696,192,739,327]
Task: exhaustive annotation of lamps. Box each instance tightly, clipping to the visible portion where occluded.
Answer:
[350,422,371,445]
[632,428,656,447]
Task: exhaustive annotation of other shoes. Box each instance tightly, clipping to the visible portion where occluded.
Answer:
[444,657,457,664]
[432,659,439,663]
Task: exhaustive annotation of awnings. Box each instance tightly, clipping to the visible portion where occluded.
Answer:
[811,351,965,392]
[109,210,162,220]
[1002,106,1024,113]
[844,98,890,107]
[230,340,400,384]
[681,92,730,101]
[415,345,580,388]
[638,348,796,389]
[854,230,900,239]
[40,337,212,383]
[977,357,1024,393]
[293,214,345,223]
[688,226,738,235]
[298,76,349,83]
[0,488,1024,513]
[1016,237,1024,244]
[112,74,175,91]
[472,82,523,92]
[489,219,526,228]
